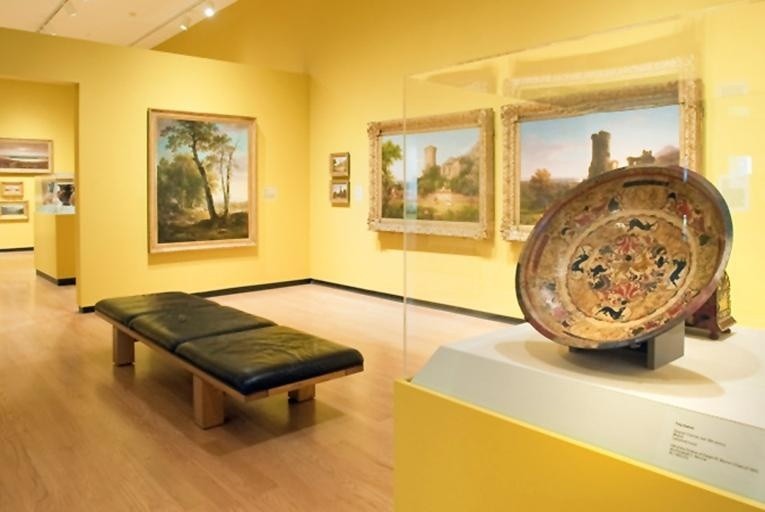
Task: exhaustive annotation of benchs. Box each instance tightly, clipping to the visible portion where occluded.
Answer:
[93,288,363,431]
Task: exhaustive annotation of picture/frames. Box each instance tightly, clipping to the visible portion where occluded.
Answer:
[147,107,261,256]
[0,139,76,223]
[329,153,349,207]
